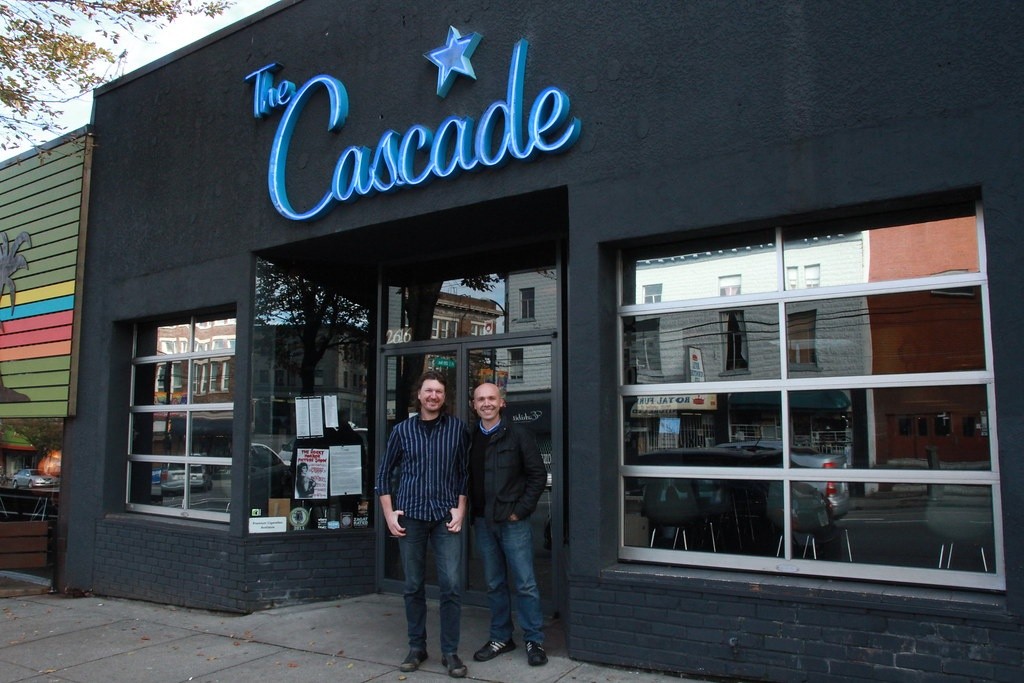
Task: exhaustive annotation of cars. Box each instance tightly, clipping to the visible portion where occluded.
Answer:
[711,439,854,520]
[278,421,369,466]
[214,443,294,502]
[539,447,835,560]
[157,451,215,494]
[11,467,59,489]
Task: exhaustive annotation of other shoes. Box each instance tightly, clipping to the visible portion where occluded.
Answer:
[400,648,429,673]
[441,654,467,678]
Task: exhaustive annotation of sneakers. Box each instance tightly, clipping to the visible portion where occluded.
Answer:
[474,639,516,662]
[525,640,548,667]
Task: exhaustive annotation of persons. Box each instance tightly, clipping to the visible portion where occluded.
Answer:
[375,371,475,679]
[469,383,550,666]
